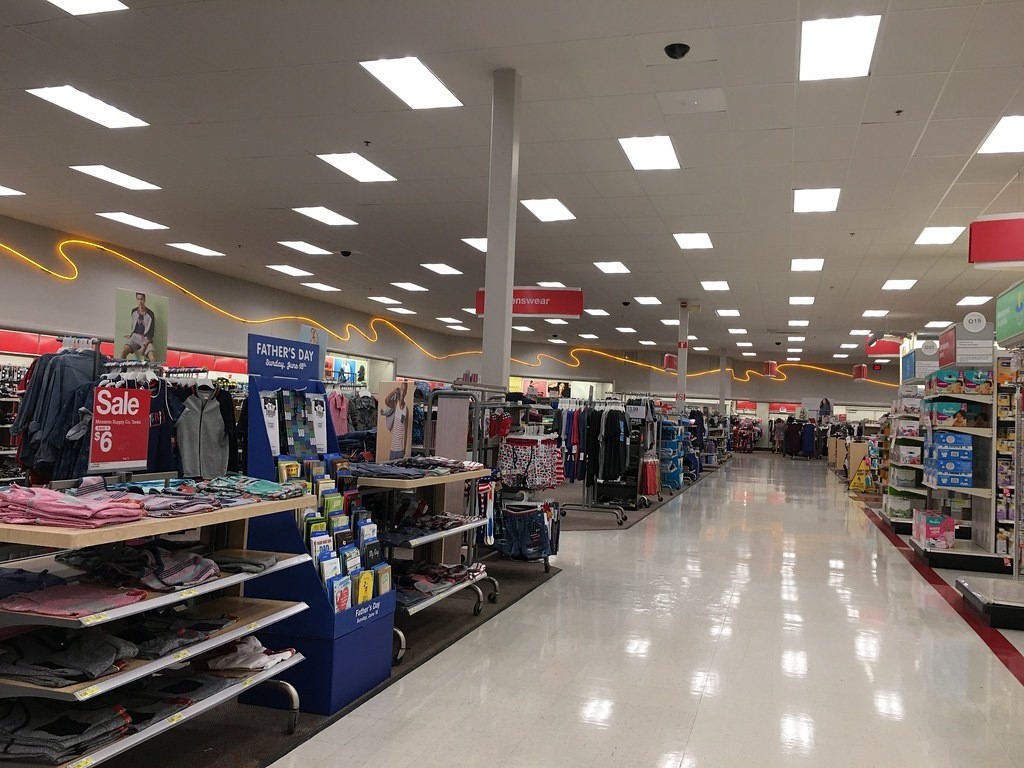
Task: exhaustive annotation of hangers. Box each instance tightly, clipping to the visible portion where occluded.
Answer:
[557,391,650,412]
[56,336,211,390]
[498,489,559,509]
[323,379,372,400]
[502,422,559,444]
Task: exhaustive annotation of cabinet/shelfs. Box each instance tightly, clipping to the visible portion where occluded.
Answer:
[0,497,317,768]
[922,347,1015,554]
[358,468,491,615]
[828,414,923,521]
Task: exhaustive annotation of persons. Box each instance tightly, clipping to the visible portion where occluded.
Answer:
[557,382,568,397]
[340,359,352,380]
[120,293,157,363]
[974,413,990,427]
[310,328,320,344]
[979,381,992,394]
[773,419,785,452]
[953,411,966,427]
[819,398,830,416]
[380,383,409,460]
[950,380,964,394]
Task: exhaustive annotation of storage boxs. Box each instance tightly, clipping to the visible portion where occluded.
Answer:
[882,369,994,549]
